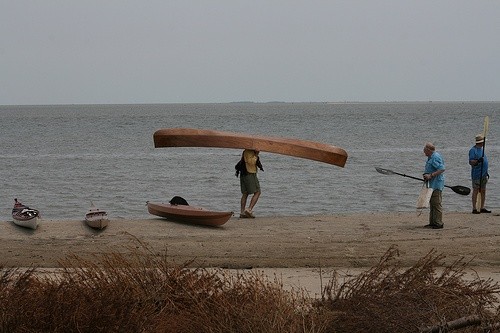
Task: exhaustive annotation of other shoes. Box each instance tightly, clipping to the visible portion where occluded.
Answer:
[472,209,480,214]
[424,224,432,227]
[480,208,491,213]
[245,210,255,218]
[432,224,443,229]
[240,214,246,218]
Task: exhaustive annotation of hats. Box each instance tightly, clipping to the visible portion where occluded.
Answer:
[475,134,488,143]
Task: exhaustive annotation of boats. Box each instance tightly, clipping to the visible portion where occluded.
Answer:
[153,129,348,168]
[144,200,235,227]
[11,198,40,231]
[84,207,110,231]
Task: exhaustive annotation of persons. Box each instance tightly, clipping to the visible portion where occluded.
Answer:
[469,134,491,214]
[423,143,446,229]
[235,149,264,218]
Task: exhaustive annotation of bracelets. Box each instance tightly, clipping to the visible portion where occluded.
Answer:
[430,173,433,178]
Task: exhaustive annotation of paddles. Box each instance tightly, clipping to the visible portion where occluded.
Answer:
[375,166,471,196]
[476,116,489,212]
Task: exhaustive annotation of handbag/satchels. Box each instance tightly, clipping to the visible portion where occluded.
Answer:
[417,173,434,209]
